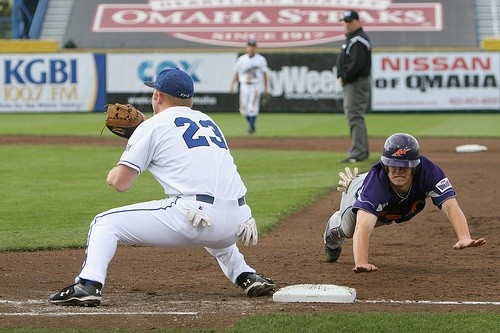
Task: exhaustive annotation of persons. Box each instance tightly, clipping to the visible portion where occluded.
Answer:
[49,68,276,307]
[336,10,372,163]
[323,133,486,274]
[230,41,268,133]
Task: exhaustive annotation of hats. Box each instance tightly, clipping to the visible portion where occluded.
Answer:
[144,68,195,99]
[341,10,360,23]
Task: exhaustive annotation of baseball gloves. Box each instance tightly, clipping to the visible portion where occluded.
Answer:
[103,102,146,139]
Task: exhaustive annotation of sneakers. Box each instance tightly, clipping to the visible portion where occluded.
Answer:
[230,270,277,297]
[49,278,102,307]
[324,247,341,261]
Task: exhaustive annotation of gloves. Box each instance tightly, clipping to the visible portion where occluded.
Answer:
[187,210,211,228]
[337,165,359,193]
[236,216,259,248]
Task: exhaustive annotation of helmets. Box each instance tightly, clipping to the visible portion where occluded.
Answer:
[381,132,420,168]
